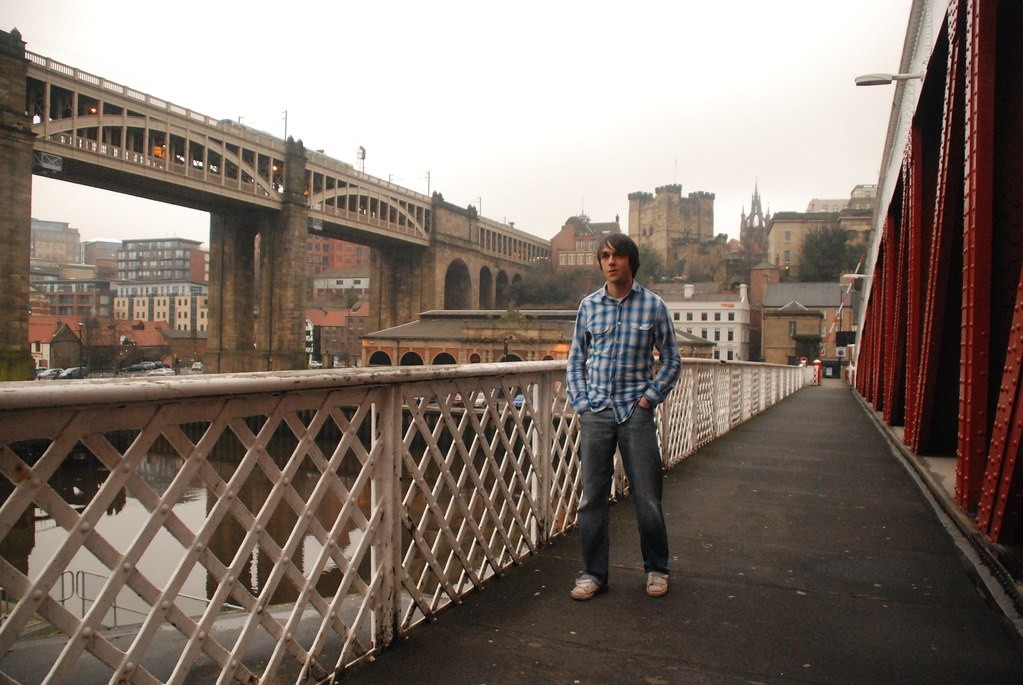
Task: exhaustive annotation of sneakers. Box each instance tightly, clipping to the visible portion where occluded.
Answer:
[571,573,601,599]
[646,571,669,596]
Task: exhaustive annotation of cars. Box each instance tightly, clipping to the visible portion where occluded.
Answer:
[191,361,203,371]
[121,359,169,372]
[35,365,88,379]
[308,360,323,369]
[147,368,175,377]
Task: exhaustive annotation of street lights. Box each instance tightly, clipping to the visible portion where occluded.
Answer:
[78,322,86,379]
[334,277,361,368]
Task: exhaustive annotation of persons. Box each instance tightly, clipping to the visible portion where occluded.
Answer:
[565,232,681,601]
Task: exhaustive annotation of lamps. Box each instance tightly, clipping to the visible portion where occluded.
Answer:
[854,70,926,87]
[842,274,875,279]
